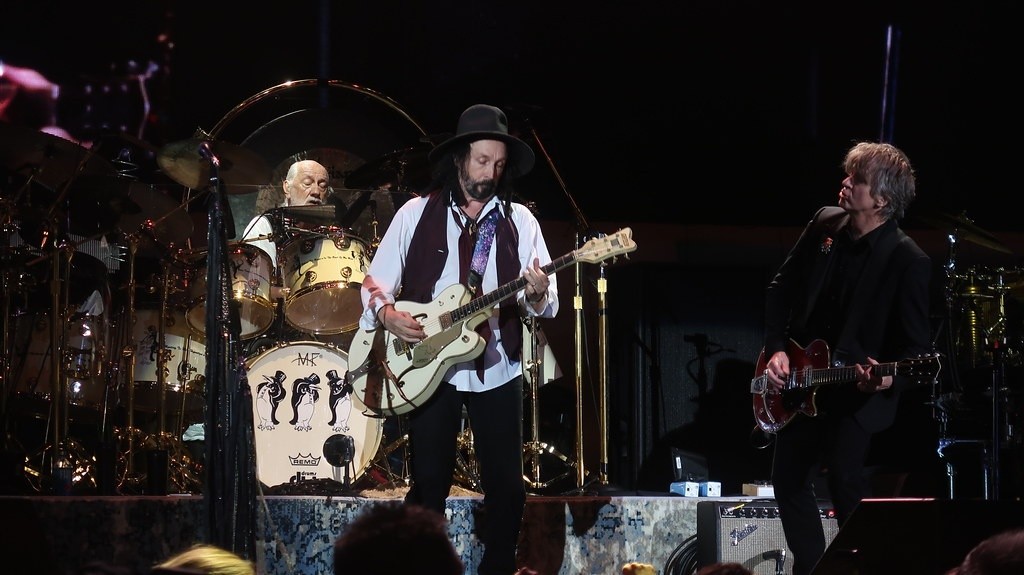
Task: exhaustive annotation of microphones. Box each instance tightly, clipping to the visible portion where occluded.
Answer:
[324,436,352,459]
[763,549,786,575]
[198,142,220,168]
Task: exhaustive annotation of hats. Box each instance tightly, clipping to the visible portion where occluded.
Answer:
[427,104,535,182]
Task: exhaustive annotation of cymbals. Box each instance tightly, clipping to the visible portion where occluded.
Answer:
[89,177,192,246]
[922,210,1016,257]
[154,135,275,195]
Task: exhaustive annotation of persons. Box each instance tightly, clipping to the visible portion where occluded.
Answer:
[335,505,466,575]
[946,529,1024,575]
[361,105,559,575]
[765,142,939,575]
[243,160,329,341]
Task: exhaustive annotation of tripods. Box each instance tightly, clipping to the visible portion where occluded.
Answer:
[517,314,597,496]
[0,164,208,496]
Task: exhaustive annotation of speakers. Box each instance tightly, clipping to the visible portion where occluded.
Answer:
[694,499,841,575]
[806,498,1024,575]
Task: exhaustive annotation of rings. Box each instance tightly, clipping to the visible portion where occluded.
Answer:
[532,283,536,286]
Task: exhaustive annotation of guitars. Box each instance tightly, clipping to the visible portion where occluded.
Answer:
[345,226,638,419]
[749,336,943,437]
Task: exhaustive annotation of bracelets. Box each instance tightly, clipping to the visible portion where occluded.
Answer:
[526,294,544,304]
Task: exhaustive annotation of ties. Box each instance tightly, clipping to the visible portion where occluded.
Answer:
[451,201,494,384]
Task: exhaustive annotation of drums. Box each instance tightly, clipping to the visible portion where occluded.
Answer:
[276,226,374,336]
[184,243,275,344]
[247,342,384,490]
[15,307,117,423]
[113,292,212,416]
[962,267,1024,370]
